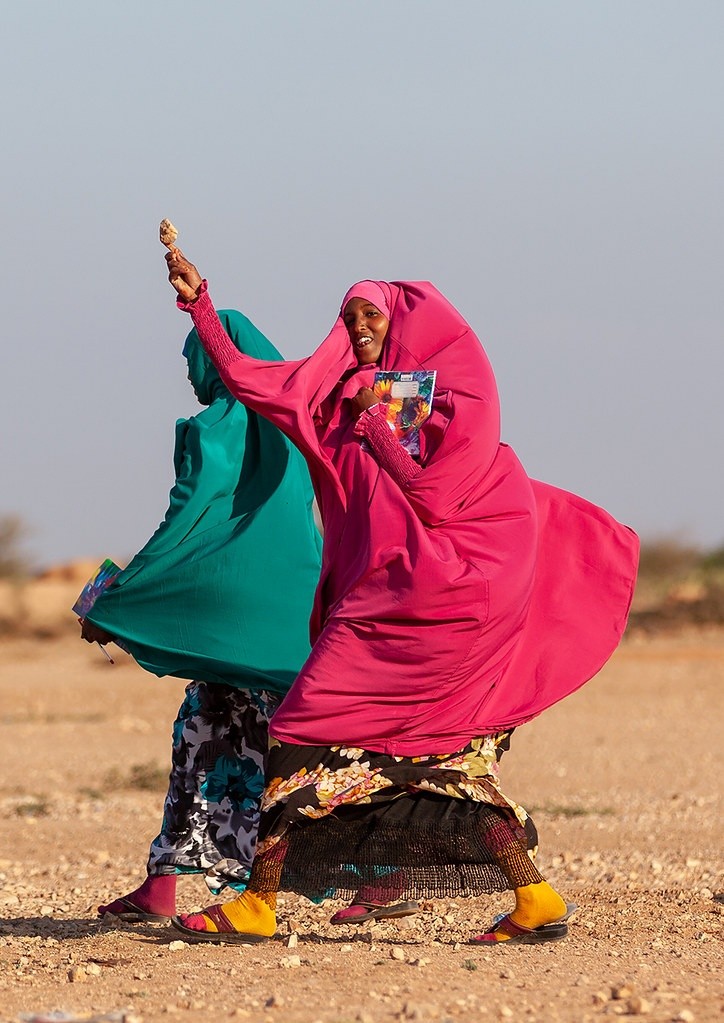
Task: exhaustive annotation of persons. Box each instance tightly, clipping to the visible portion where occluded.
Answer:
[164,248,642,947]
[80,307,418,925]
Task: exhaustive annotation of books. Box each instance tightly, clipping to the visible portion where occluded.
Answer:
[72,558,130,655]
[360,369,438,456]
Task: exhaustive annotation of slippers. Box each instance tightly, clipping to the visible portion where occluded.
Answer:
[331,901,418,925]
[97,898,171,922]
[171,904,268,945]
[469,914,568,946]
